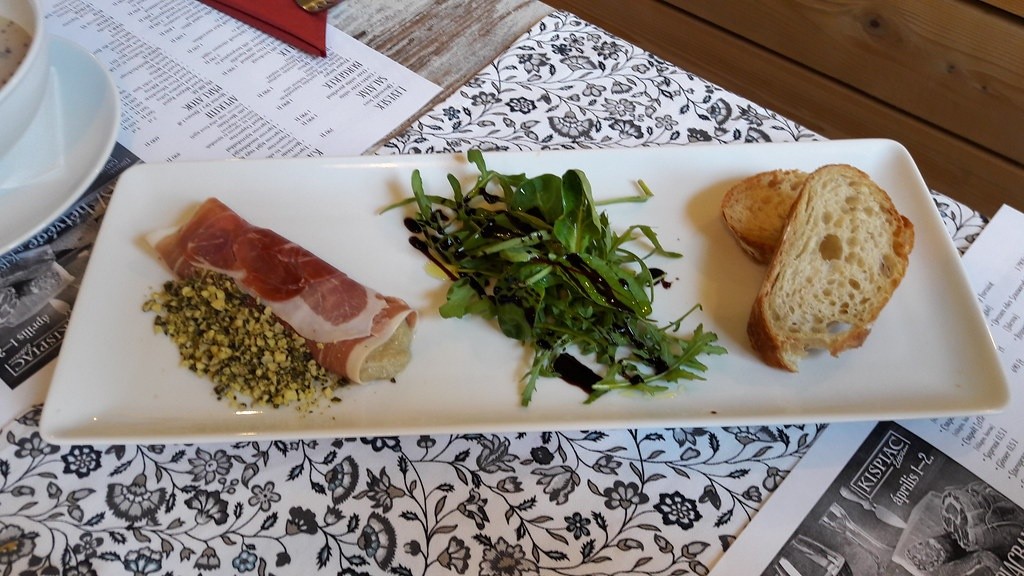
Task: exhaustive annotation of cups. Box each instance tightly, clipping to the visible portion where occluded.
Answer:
[0,0,50,159]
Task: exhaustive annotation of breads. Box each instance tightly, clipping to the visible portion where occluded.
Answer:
[749,162,914,372]
[726,170,806,262]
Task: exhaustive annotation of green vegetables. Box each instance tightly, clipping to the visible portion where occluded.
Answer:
[378,150,726,405]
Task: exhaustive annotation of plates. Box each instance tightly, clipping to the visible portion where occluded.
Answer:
[39,138,1012,445]
[0,34,120,255]
